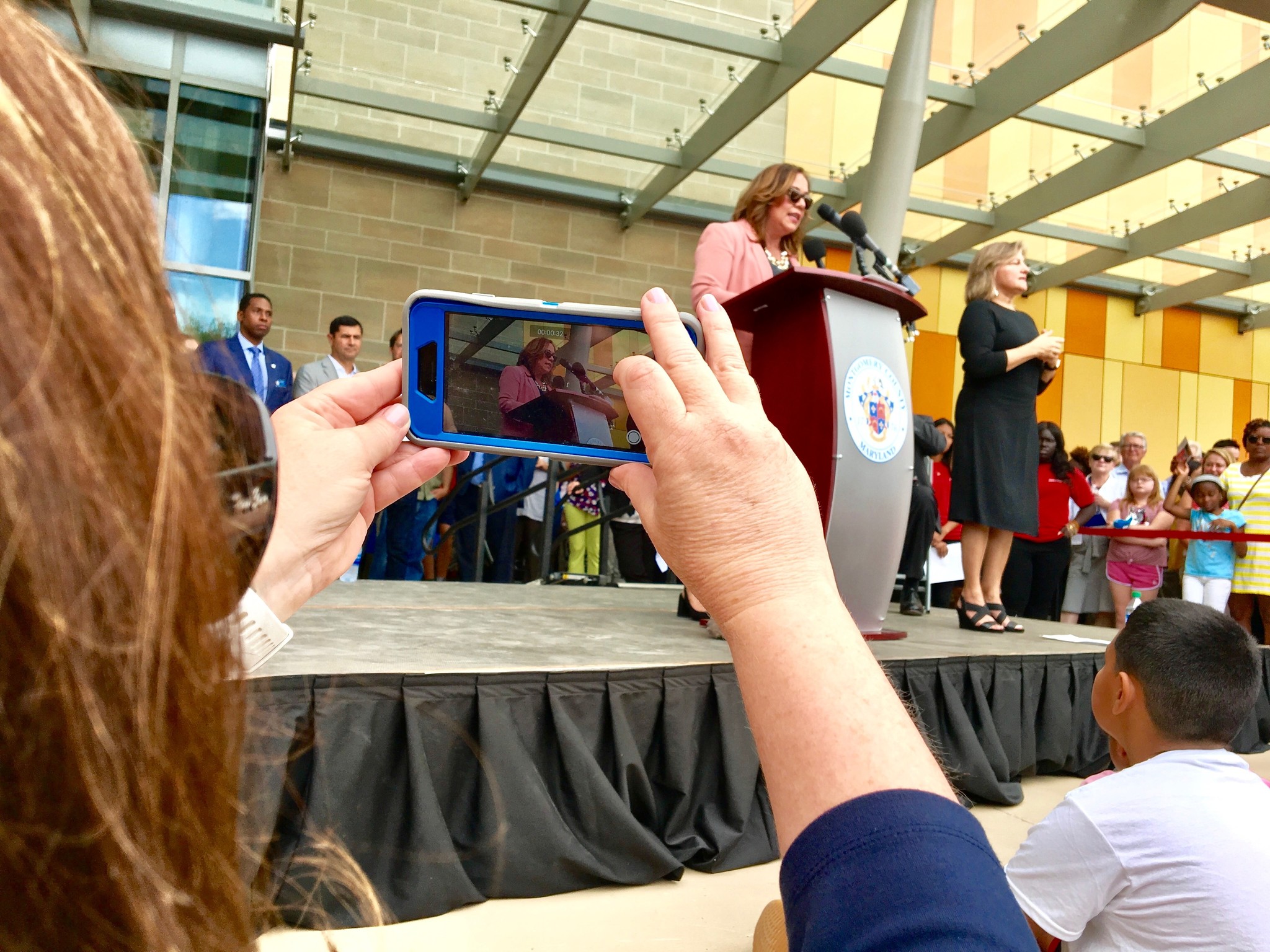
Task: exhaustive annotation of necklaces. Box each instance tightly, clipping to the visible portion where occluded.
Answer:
[762,241,790,271]
[532,374,547,392]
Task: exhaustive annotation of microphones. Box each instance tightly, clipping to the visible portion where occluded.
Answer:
[843,212,887,267]
[553,358,605,400]
[816,203,868,251]
[801,238,826,268]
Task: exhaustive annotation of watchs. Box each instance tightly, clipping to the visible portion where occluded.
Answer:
[1043,358,1061,372]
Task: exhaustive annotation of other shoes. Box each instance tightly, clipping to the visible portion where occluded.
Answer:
[677,588,710,621]
[900,588,924,615]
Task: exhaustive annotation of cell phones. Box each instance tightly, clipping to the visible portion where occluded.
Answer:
[402,290,706,467]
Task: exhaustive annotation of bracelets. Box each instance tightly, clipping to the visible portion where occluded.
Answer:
[1064,519,1080,537]
[207,588,294,673]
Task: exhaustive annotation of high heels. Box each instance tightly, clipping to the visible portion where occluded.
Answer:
[955,595,1005,632]
[986,603,1025,632]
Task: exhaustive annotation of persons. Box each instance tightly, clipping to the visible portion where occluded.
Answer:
[293,314,364,401]
[193,293,295,418]
[1217,418,1270,647]
[498,337,558,439]
[949,239,1065,633]
[690,163,813,377]
[1001,422,1099,624]
[898,413,964,616]
[0,1,1051,952]
[1107,464,1175,631]
[344,322,605,579]
[1064,433,1240,522]
[754,596,1270,952]
[1162,459,1248,614]
[1060,445,1126,627]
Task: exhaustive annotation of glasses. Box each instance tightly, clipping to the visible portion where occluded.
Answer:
[195,367,279,500]
[1092,454,1114,462]
[1248,434,1270,444]
[544,350,558,362]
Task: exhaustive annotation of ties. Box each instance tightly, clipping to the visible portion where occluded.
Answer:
[249,347,265,401]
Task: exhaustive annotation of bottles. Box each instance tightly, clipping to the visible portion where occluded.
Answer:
[339,546,363,582]
[1125,591,1143,624]
